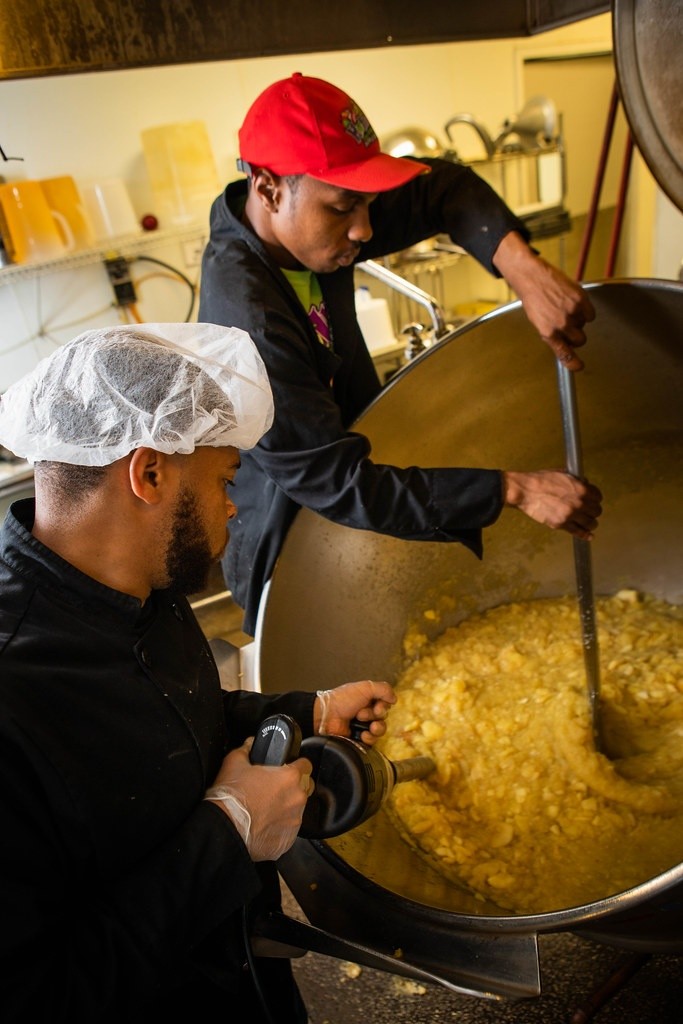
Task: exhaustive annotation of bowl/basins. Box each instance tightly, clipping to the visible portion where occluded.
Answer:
[243,276,682,934]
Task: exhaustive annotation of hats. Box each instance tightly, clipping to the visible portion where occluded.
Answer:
[237,72,432,193]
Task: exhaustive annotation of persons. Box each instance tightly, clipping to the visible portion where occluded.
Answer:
[199,71,605,640]
[0,321,398,1024]
